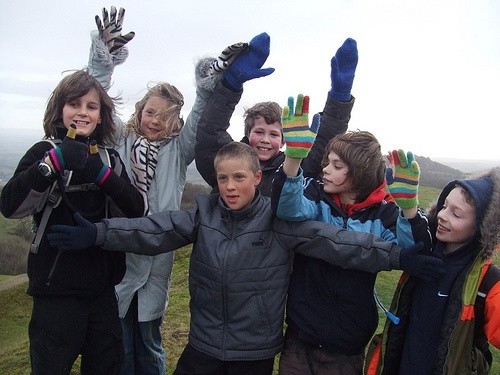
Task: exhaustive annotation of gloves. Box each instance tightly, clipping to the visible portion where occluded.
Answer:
[224,32,276,91]
[66,139,112,187]
[281,93,320,158]
[399,241,447,286]
[48,211,96,251]
[212,42,249,73]
[329,37,359,103]
[49,123,90,174]
[384,149,421,211]
[94,5,136,54]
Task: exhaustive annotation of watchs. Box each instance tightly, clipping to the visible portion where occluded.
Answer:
[38,158,55,177]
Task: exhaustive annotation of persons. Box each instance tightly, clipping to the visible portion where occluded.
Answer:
[0,6,499,375]
